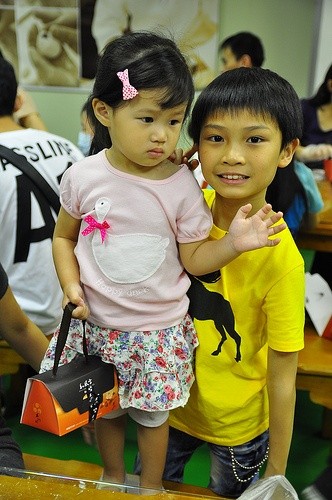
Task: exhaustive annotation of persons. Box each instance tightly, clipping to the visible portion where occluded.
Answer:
[52,31,286,496]
[131,66,305,500]
[0,261,51,476]
[0,30,332,500]
[0,50,85,341]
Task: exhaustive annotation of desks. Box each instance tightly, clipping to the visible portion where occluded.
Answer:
[297,177,332,253]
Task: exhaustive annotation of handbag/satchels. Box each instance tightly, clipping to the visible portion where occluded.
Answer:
[20,301,119,436]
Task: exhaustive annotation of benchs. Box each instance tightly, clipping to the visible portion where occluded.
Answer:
[295,323,332,440]
